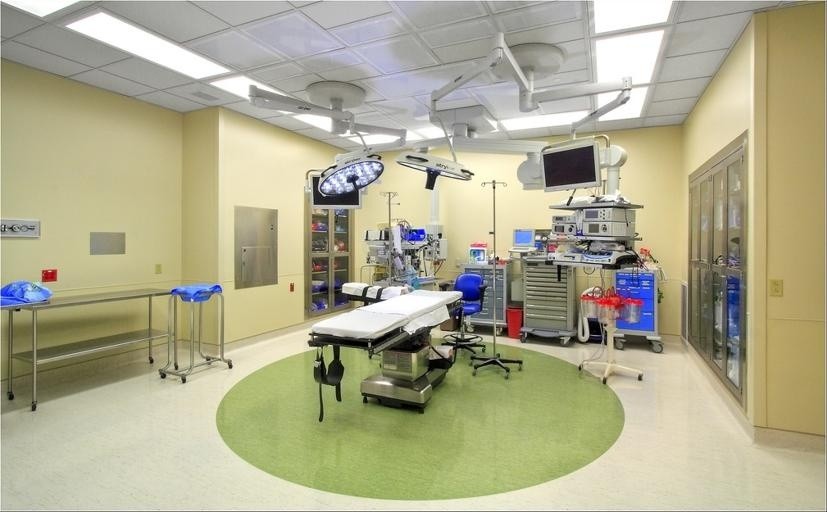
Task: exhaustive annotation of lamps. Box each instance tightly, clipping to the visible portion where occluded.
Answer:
[318,118,386,197]
[397,117,475,190]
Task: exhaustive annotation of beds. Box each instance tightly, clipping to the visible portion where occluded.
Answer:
[342,282,407,304]
[308,290,463,414]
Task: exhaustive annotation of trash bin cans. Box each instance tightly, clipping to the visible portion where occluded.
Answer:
[506,305,523,339]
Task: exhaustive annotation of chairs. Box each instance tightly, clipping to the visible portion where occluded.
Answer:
[439,273,487,355]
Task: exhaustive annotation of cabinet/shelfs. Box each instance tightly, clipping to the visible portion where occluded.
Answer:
[7,288,179,411]
[308,209,353,317]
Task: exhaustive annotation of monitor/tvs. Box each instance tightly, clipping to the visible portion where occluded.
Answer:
[310,173,361,208]
[541,141,601,192]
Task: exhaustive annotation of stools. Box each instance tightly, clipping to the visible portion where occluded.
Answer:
[158,284,233,383]
